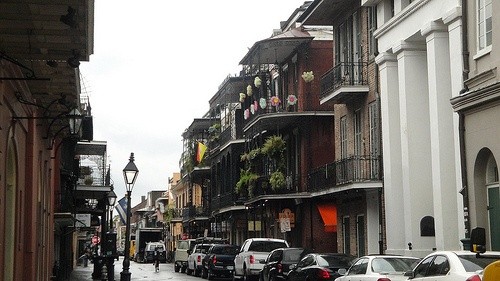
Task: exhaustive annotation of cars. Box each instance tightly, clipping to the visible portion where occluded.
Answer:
[404,250,500,281]
[259,248,315,281]
[286,253,368,281]
[335,254,423,281]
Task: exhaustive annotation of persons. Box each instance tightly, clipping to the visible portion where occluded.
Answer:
[130,247,173,270]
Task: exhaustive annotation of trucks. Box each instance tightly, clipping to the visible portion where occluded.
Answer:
[117,225,163,263]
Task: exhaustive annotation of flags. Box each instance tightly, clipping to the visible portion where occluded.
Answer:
[115,196,128,225]
[197,141,207,164]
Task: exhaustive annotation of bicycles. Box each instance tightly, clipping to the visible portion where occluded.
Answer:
[153,258,159,272]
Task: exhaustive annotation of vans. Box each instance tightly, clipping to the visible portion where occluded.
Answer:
[143,240,167,264]
[173,238,196,273]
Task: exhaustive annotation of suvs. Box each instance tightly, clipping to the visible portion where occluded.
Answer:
[231,238,290,281]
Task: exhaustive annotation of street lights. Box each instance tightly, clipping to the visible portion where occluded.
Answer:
[107,185,117,255]
[121,153,139,281]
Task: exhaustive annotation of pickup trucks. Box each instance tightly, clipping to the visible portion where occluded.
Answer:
[186,244,214,276]
[202,244,241,280]
